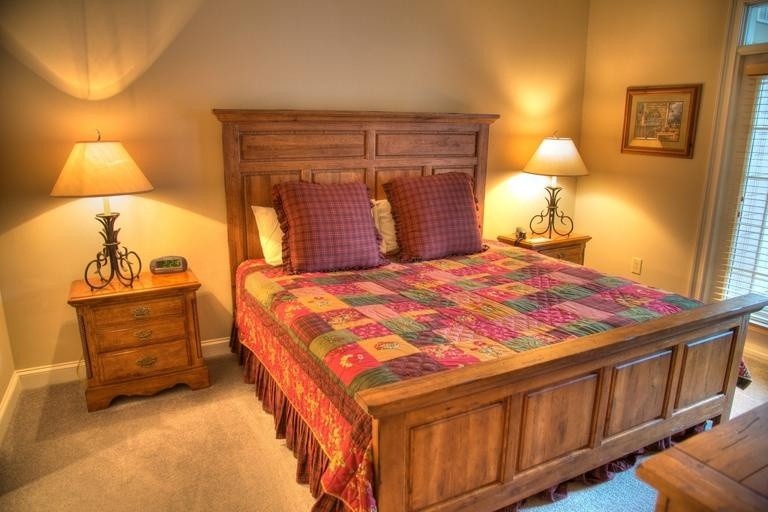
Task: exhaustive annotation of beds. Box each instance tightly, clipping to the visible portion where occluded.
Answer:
[212,108,768,512]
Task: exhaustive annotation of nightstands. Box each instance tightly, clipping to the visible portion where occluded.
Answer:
[67,268,210,412]
[498,230,593,266]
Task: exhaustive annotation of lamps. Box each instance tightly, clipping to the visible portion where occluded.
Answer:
[522,131,590,239]
[50,131,155,290]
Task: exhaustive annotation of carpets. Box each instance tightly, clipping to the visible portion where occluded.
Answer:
[515,420,716,512]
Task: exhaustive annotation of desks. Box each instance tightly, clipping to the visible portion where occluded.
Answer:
[637,403,767,512]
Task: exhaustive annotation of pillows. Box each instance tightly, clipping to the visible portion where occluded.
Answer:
[382,172,489,263]
[250,199,387,267]
[272,180,391,275]
[375,198,401,256]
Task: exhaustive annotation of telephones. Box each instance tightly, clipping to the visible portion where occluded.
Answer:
[516,227,526,240]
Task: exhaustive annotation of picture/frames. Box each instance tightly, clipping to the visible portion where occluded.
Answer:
[621,84,702,159]
